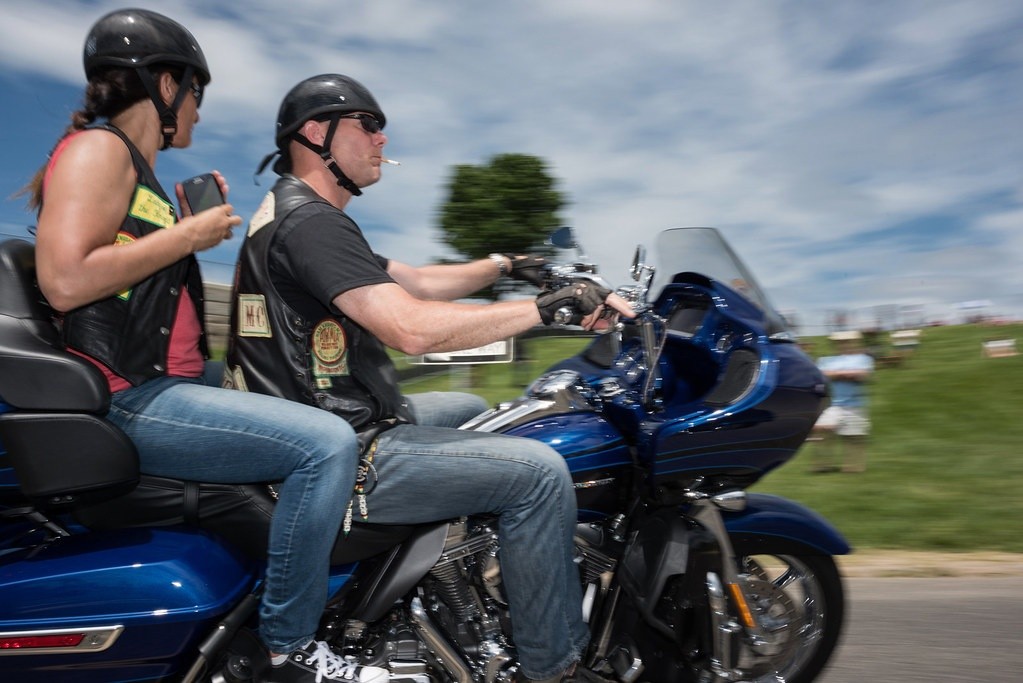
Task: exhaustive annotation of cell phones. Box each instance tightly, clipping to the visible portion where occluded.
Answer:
[182,173,225,216]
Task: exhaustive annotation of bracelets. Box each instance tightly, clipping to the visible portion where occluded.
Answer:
[488,252,509,277]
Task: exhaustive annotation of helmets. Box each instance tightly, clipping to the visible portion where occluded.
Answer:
[275,74,385,141]
[84,9,211,88]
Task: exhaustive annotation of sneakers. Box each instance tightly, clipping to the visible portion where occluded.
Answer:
[268,639,390,683]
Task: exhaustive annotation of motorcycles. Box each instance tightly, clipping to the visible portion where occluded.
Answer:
[0,223,854,683]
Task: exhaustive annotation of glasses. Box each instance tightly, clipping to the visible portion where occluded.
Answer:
[314,113,383,133]
[175,74,204,108]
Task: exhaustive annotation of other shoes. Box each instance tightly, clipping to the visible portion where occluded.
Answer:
[511,662,616,683]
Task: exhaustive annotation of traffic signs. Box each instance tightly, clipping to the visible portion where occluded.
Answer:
[424,333,515,365]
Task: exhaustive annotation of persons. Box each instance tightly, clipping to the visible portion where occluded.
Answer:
[223,73,637,683]
[10,7,393,683]
[809,330,873,475]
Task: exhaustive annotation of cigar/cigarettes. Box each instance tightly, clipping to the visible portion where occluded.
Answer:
[379,156,401,165]
